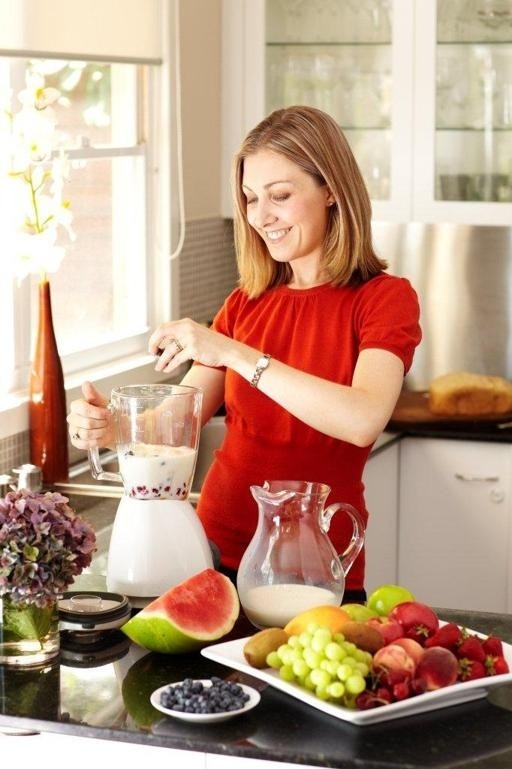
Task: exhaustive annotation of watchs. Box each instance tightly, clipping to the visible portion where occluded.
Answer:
[250,353,273,388]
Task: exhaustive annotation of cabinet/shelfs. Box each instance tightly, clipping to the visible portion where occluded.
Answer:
[219,1,512,231]
[361,441,400,600]
[399,437,510,619]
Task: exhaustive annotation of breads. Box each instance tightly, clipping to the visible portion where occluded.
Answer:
[428,371,512,417]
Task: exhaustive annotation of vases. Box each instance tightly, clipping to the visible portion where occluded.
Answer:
[30,281,66,486]
[0,602,61,671]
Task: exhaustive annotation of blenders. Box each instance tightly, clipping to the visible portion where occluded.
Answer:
[86,384,213,597]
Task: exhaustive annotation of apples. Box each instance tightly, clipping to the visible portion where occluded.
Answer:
[341,586,415,622]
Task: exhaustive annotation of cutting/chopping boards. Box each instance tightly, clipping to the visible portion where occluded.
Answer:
[385,390,512,430]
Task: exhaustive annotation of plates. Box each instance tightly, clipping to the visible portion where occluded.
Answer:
[201,620,512,728]
[151,678,260,724]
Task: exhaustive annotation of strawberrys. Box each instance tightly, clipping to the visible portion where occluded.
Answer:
[422,623,510,683]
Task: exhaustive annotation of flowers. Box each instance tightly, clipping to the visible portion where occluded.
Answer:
[0,490,97,647]
[4,85,68,278]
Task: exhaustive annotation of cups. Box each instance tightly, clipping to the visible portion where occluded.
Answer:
[268,52,389,128]
[433,173,512,202]
[435,61,512,128]
[265,2,392,43]
[437,1,512,43]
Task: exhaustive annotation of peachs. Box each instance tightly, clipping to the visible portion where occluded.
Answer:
[366,602,460,692]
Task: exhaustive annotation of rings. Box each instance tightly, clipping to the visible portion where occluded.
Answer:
[171,339,183,352]
[71,427,82,442]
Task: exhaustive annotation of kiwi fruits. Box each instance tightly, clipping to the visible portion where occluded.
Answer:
[336,623,385,654]
[244,628,291,670]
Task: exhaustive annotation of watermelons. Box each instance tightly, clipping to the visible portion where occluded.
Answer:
[119,568,241,653]
[121,650,237,730]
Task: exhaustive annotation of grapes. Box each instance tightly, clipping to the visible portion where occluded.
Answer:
[265,623,372,708]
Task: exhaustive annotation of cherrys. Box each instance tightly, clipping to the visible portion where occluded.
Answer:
[356,673,411,710]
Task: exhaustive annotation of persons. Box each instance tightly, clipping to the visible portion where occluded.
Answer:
[64,104,424,607]
